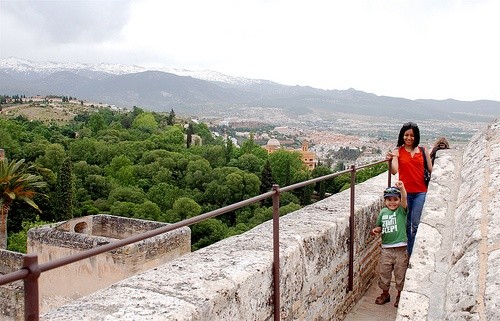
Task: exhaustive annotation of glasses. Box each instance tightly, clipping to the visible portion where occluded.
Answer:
[403,122,417,127]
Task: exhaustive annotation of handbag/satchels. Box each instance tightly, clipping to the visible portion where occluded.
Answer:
[421,147,431,187]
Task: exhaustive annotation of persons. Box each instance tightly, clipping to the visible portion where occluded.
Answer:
[385,122,433,259]
[430,137,449,166]
[371,181,408,307]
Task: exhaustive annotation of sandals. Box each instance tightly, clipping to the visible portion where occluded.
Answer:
[375,293,390,305]
[394,297,399,307]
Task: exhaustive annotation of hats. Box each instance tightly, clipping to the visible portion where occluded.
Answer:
[383,187,401,197]
